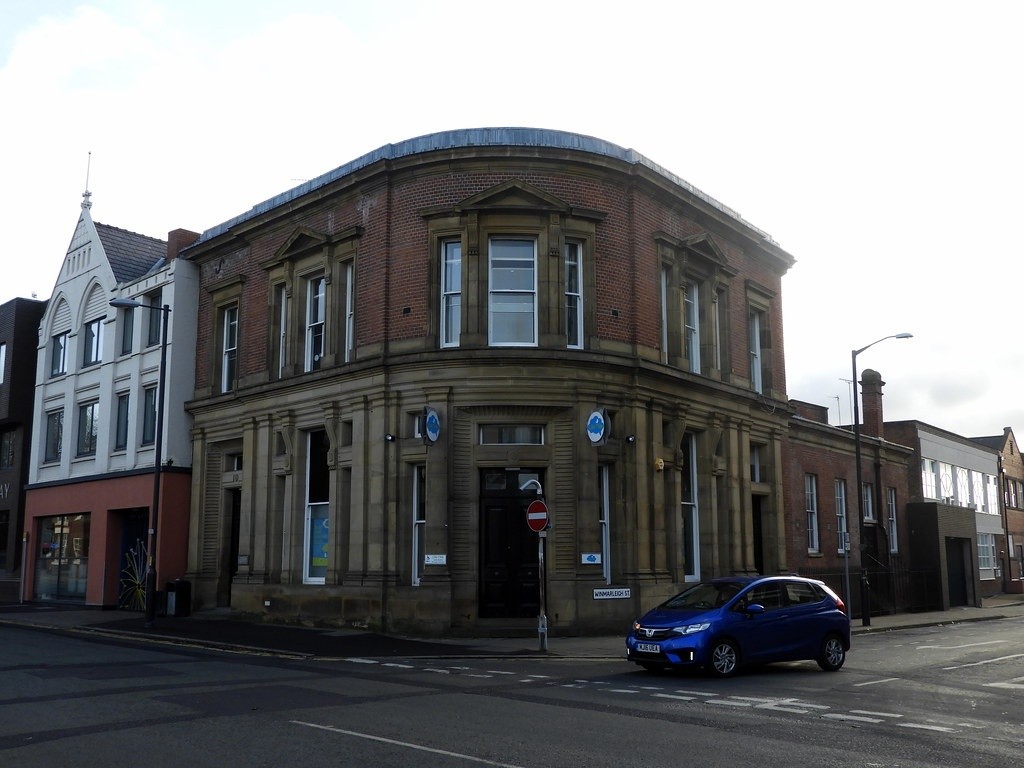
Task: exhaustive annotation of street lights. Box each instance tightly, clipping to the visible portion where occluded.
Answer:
[851,332,914,627]
[108,298,173,630]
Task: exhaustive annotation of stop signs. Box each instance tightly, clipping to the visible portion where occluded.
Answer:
[526,500,548,533]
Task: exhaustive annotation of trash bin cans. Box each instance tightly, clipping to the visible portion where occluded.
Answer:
[165,579,192,617]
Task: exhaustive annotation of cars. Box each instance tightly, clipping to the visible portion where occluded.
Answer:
[624,573,850,678]
